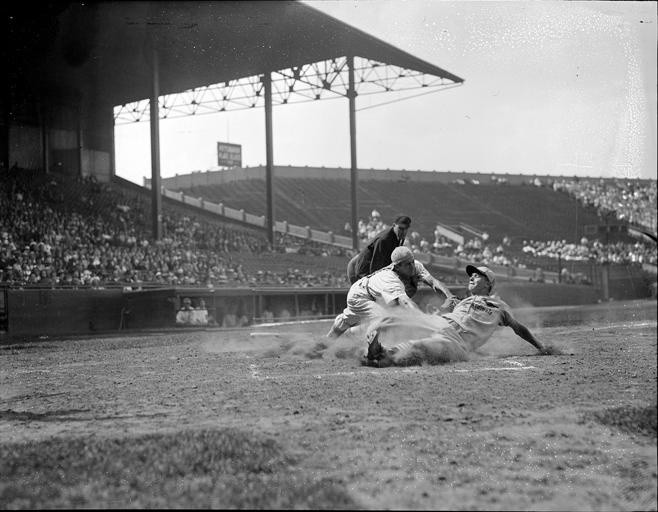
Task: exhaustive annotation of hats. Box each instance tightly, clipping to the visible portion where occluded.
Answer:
[391,246,415,270]
[466,264,496,290]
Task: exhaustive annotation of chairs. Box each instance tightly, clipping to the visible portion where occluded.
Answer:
[0,178,658,300]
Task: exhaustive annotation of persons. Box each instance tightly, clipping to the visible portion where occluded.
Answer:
[299,243,460,359]
[360,265,564,367]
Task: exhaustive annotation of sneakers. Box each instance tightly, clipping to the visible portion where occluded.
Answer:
[363,330,385,361]
